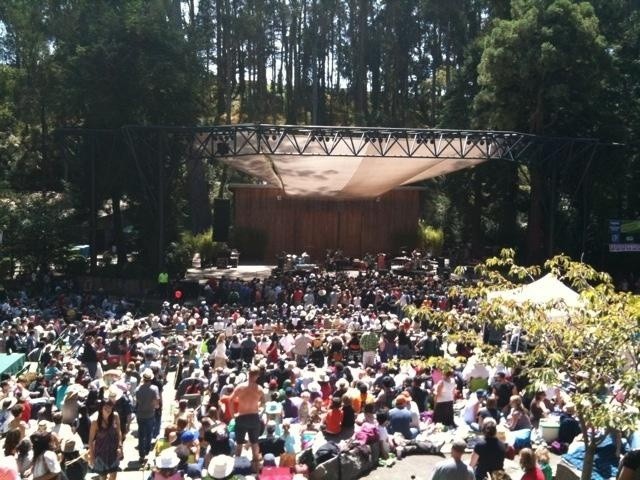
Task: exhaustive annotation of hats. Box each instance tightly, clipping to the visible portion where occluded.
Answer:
[155,448,181,468]
[104,384,123,401]
[207,453,236,479]
[37,419,55,432]
[60,432,84,453]
[179,430,199,442]
[0,396,17,410]
[140,367,155,381]
[308,369,349,393]
[63,383,84,402]
[191,368,204,378]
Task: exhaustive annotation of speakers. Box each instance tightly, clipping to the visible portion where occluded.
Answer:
[212,197,230,243]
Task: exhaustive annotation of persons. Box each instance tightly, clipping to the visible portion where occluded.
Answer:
[0,248,640,479]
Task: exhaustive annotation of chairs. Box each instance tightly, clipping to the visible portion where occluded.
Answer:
[282,255,440,277]
